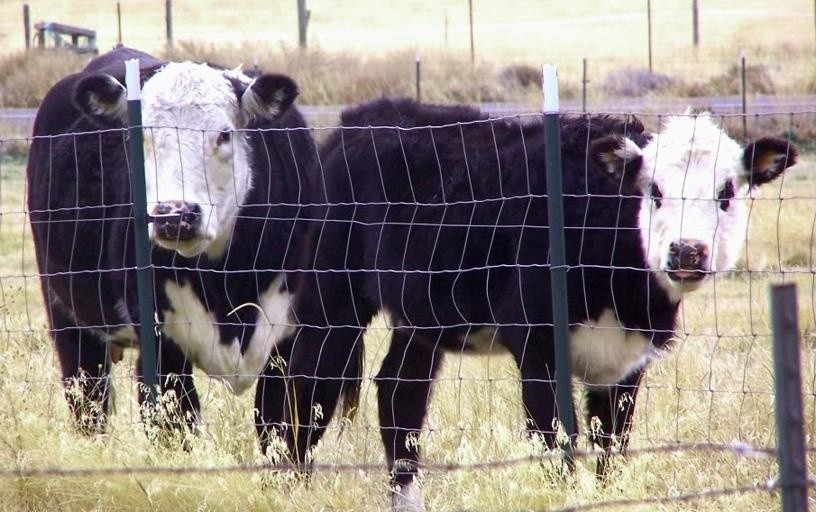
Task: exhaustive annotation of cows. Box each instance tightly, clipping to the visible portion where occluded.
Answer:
[24,46,318,455]
[285,97,799,512]
